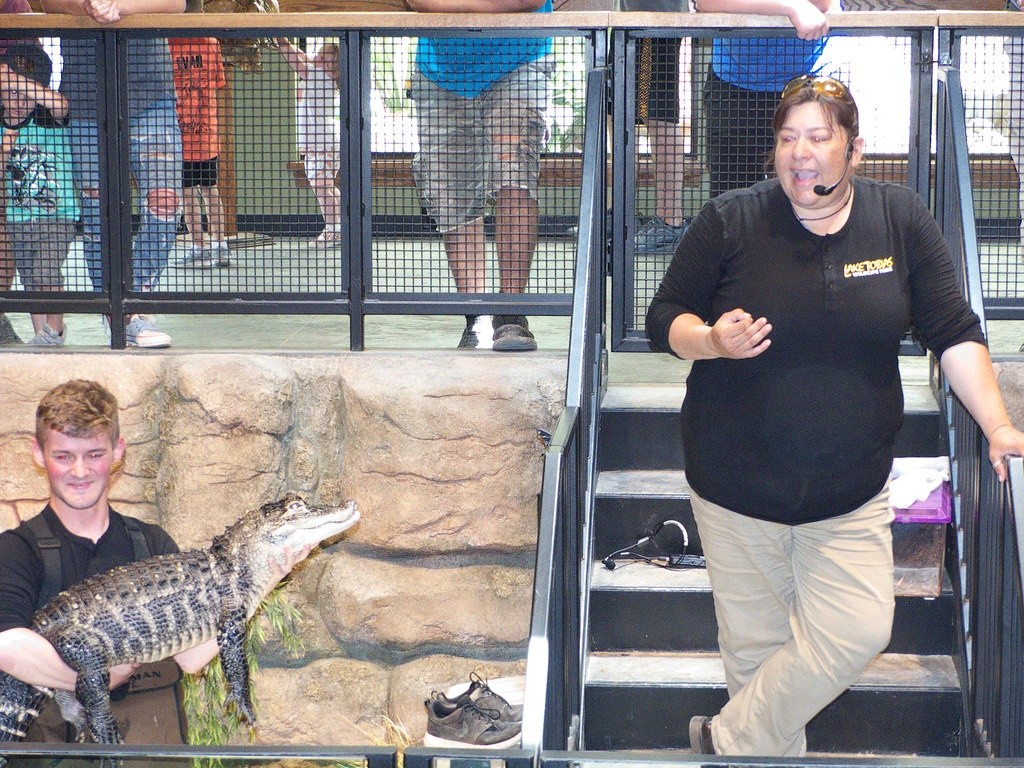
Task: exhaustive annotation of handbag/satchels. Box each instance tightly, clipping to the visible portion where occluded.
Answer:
[22,657,193,768]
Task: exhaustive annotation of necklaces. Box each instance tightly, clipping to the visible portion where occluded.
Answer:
[789,179,853,223]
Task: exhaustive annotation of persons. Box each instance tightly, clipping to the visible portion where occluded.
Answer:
[566,0,690,259]
[643,75,1024,768]
[0,0,82,346]
[277,36,341,248]
[40,0,187,348]
[0,379,221,768]
[690,0,841,200]
[167,37,231,269]
[400,0,553,350]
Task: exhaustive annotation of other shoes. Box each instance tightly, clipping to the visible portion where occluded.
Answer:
[689,714,731,768]
[313,224,342,244]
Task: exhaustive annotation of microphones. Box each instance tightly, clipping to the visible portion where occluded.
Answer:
[813,159,850,196]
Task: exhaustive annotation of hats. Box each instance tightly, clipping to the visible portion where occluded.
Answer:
[1,41,53,88]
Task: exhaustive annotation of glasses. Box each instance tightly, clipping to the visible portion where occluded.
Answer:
[781,75,857,127]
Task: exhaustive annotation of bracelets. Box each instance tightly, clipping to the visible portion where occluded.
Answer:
[987,424,1011,442]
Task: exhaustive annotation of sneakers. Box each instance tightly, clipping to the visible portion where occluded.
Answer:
[208,245,232,268]
[173,246,213,269]
[457,314,494,349]
[491,313,538,351]
[565,206,644,240]
[423,698,522,751]
[28,321,69,346]
[634,214,694,254]
[431,672,524,723]
[125,313,173,348]
